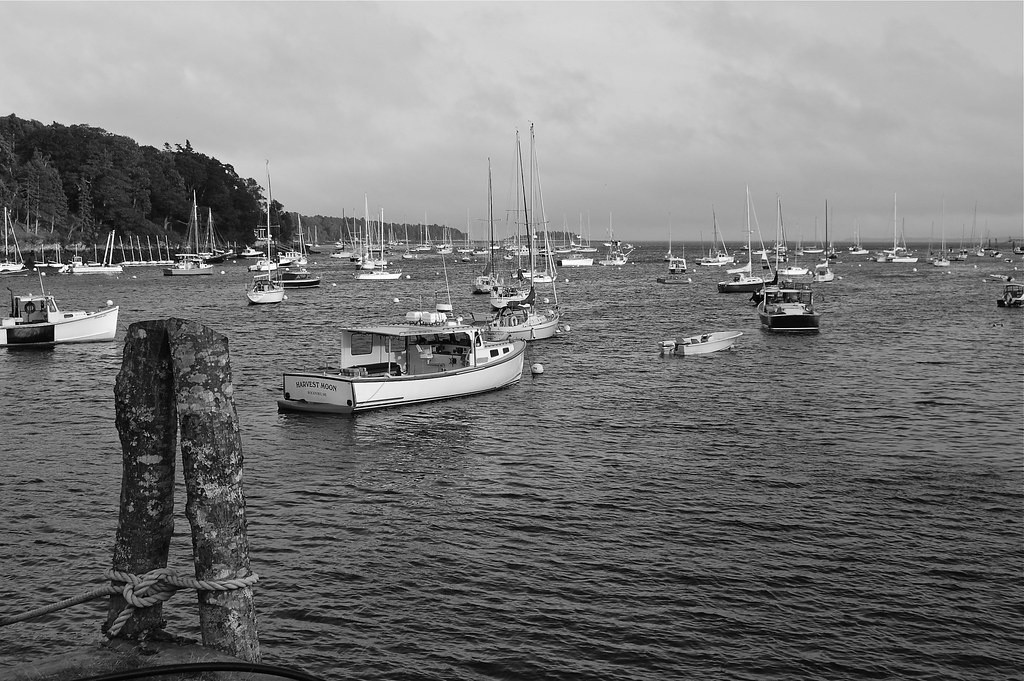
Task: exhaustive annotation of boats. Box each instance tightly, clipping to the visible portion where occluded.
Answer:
[736,244,842,263]
[472,275,505,294]
[669,258,688,273]
[757,279,821,335]
[0,292,119,347]
[474,307,561,342]
[725,263,753,274]
[657,331,743,356]
[277,303,529,415]
[717,272,764,293]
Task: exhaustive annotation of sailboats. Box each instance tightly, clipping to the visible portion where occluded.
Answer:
[849,192,1024,267]
[328,192,404,281]
[402,208,597,268]
[510,131,538,279]
[813,199,834,282]
[603,212,621,247]
[692,205,736,264]
[739,186,778,285]
[490,285,537,309]
[521,123,558,284]
[0,160,320,306]
[663,213,678,262]
[771,194,809,276]
[598,229,628,266]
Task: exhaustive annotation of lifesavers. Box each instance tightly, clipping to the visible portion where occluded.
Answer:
[509,316,518,325]
[24,302,36,313]
